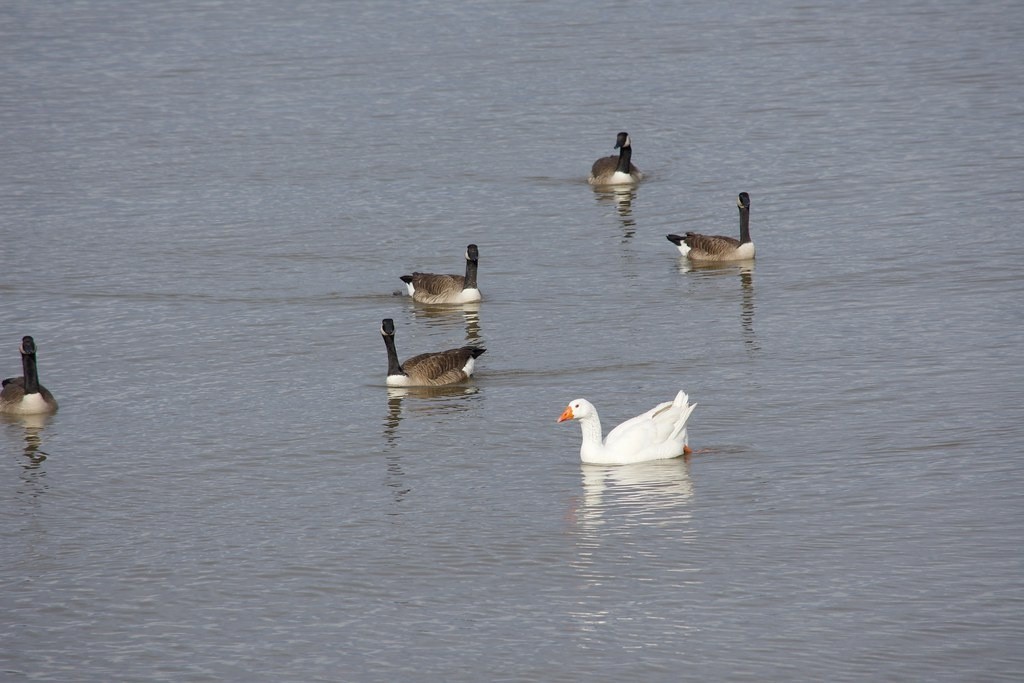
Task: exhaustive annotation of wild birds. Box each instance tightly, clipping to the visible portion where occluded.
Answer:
[587,132,644,186]
[380,318,487,387]
[664,192,756,261]
[398,244,482,306]
[0,336,59,414]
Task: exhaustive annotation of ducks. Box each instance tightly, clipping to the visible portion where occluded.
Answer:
[557,389,712,465]
